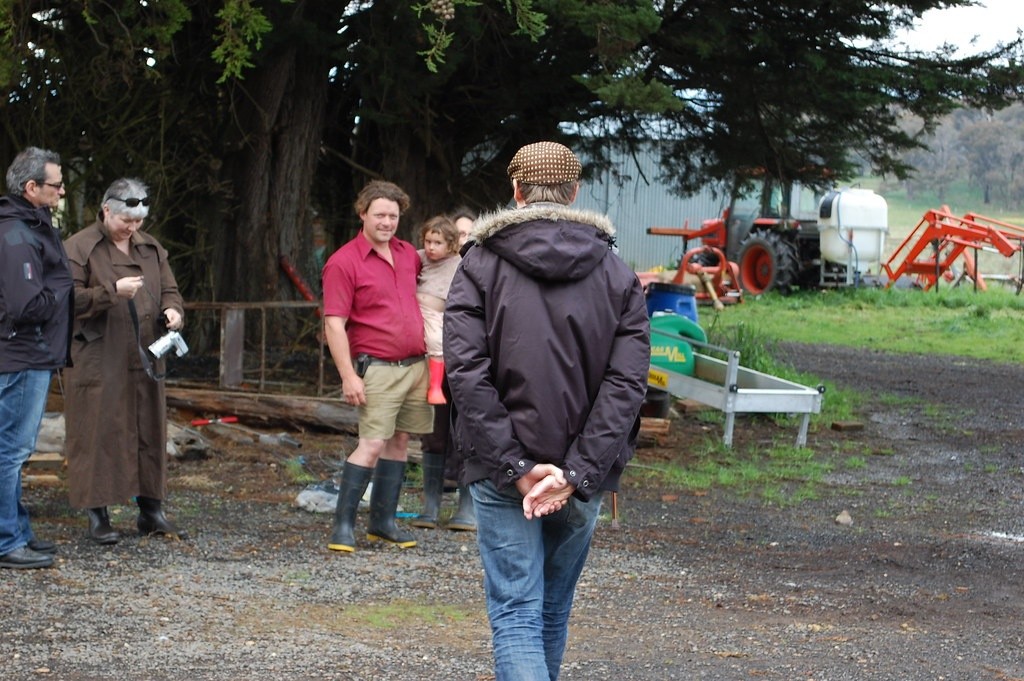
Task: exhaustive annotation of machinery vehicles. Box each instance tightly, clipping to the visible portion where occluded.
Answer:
[639,168,890,303]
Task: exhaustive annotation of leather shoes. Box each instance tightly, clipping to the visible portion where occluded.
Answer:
[0,546,55,570]
[28,537,57,554]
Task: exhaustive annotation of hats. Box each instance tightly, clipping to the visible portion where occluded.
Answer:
[506,142,583,186]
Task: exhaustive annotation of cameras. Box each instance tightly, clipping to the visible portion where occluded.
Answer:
[148,331,189,359]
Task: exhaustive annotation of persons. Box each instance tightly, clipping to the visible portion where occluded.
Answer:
[58,178,184,541]
[323,179,434,552]
[443,142,651,681]
[0,147,75,568]
[416,217,463,404]
[410,211,479,530]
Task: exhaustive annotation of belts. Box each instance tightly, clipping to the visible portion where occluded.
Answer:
[351,354,426,366]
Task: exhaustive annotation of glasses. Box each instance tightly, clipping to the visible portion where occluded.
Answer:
[105,196,153,207]
[37,179,65,190]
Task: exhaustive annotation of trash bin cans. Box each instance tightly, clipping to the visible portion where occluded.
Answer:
[645,282,698,324]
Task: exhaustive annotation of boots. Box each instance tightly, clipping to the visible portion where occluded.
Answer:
[410,452,447,529]
[427,356,447,405]
[446,464,477,531]
[326,459,376,552]
[86,505,123,545]
[135,495,189,539]
[366,456,417,548]
